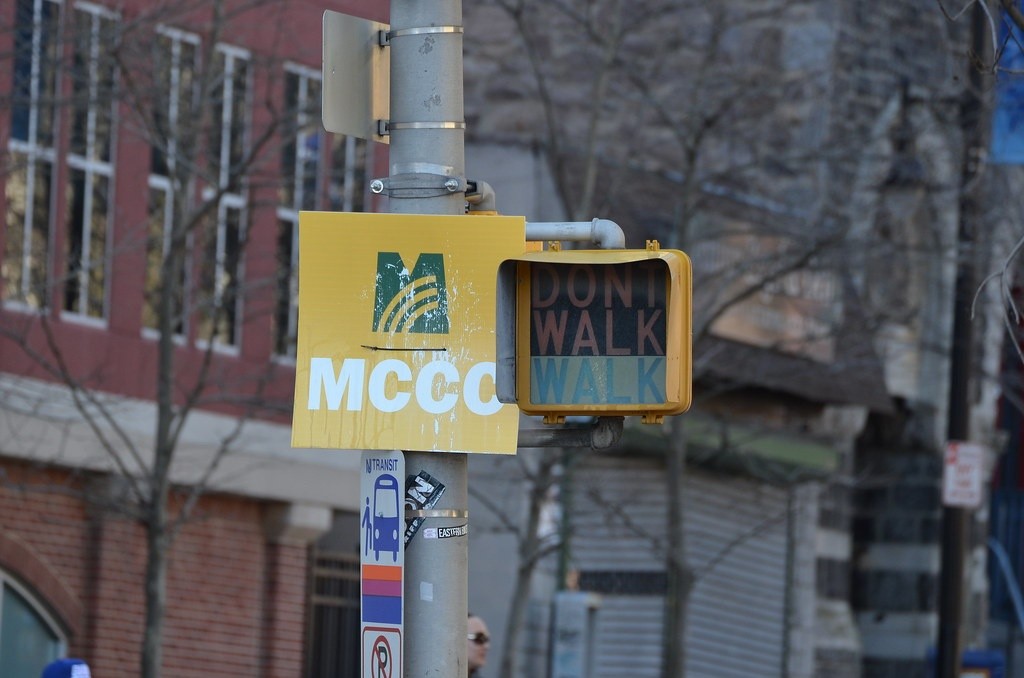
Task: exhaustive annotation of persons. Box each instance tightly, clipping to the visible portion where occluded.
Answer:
[467,613,490,678]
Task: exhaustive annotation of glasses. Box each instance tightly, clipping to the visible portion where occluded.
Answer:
[467,633,490,645]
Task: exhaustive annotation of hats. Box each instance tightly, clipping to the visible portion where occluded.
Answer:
[42,657,91,678]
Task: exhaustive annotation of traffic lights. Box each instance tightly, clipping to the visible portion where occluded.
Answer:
[493,244,692,426]
